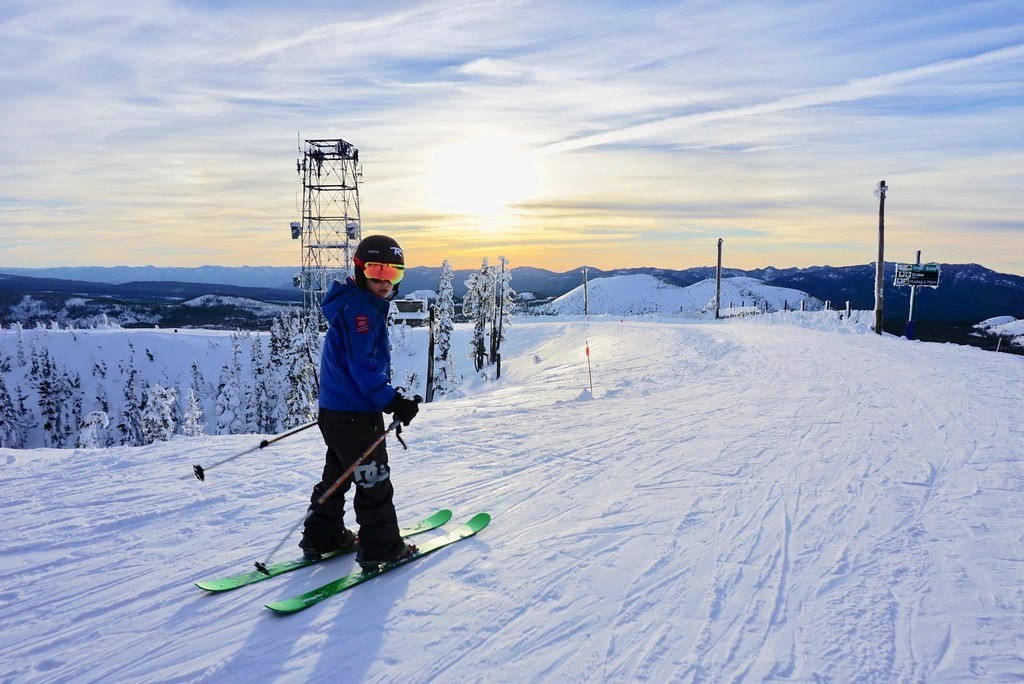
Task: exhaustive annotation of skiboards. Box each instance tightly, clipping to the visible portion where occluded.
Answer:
[194,509,496,613]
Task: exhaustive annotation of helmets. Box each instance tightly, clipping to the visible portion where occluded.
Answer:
[353,234,405,286]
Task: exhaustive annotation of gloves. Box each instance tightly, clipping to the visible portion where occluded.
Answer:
[383,393,419,450]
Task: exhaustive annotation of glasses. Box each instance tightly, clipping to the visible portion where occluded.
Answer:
[355,256,405,285]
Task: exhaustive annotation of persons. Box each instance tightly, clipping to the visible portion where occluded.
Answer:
[300,235,419,572]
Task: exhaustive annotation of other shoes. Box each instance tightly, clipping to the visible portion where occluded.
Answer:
[354,542,417,573]
[298,529,357,561]
